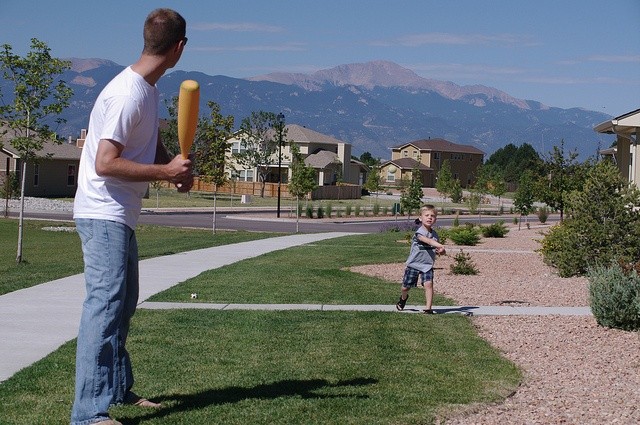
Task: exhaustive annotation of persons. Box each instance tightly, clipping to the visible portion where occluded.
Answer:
[396,204,445,313]
[70,7,194,425]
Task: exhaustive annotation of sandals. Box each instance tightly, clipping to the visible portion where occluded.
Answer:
[420,309,432,314]
[396,294,409,311]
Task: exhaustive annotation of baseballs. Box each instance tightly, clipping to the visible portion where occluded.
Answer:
[190,293,197,299]
[387,165,397,174]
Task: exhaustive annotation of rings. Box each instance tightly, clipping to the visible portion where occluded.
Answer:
[176,183,182,188]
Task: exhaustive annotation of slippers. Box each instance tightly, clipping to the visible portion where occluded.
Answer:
[134,398,161,407]
[89,420,122,424]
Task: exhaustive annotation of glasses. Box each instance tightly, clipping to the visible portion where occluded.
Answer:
[179,37,189,45]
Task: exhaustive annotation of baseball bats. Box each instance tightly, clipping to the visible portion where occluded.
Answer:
[177,80,201,159]
